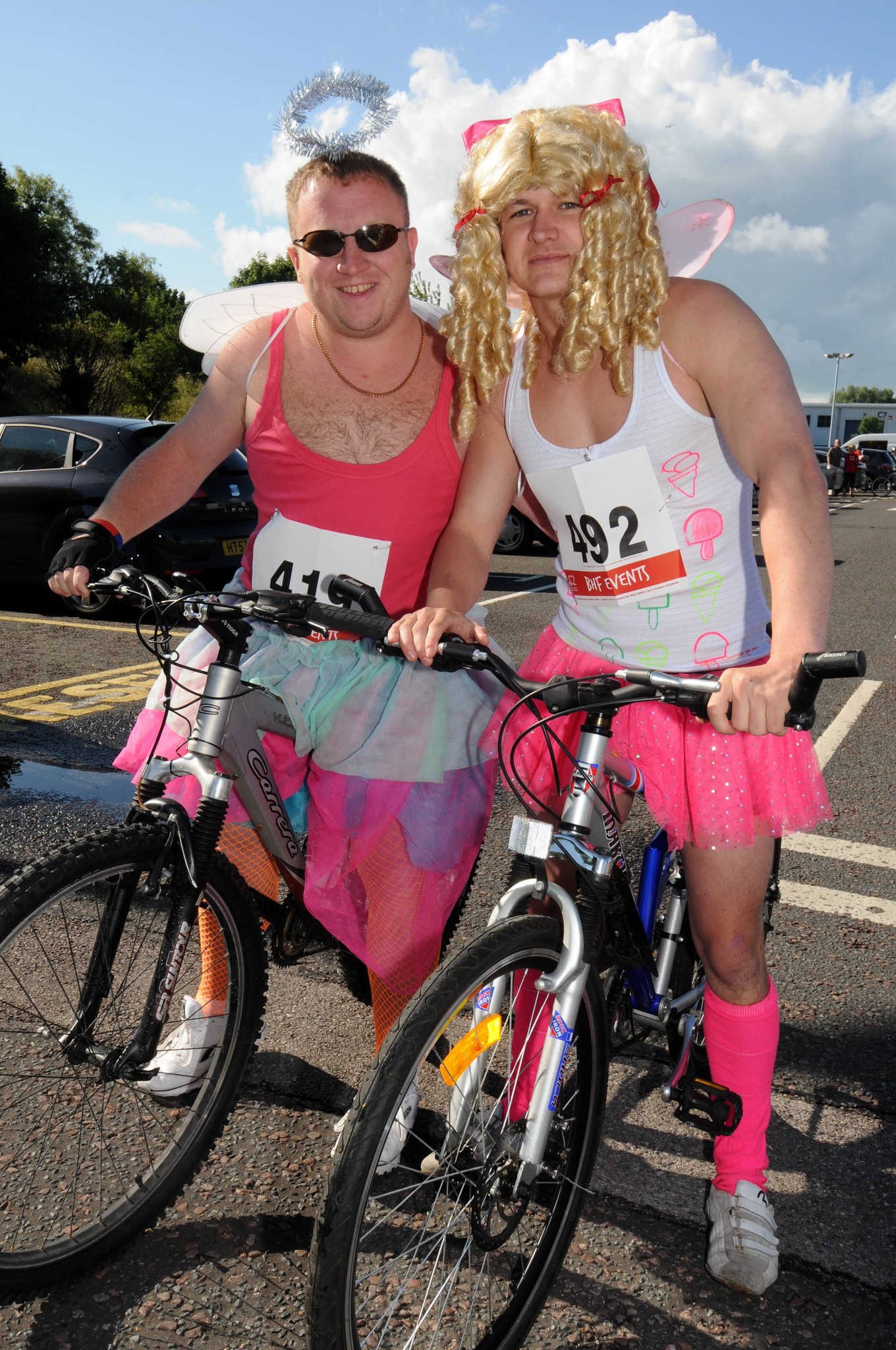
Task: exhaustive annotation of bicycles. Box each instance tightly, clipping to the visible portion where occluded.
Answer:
[306,568,870,1349]
[871,465,896,497]
[0,561,501,1288]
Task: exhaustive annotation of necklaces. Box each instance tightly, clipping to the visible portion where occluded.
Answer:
[311,313,425,398]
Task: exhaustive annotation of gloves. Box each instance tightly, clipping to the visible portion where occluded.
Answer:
[827,465,831,469]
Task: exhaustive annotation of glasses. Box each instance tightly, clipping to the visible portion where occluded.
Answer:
[292,223,410,257]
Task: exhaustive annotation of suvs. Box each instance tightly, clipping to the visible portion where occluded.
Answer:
[1,415,258,621]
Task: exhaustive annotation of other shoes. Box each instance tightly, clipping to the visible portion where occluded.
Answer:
[849,494,854,497]
[842,493,846,497]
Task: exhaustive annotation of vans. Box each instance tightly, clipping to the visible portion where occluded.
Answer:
[840,433,896,463]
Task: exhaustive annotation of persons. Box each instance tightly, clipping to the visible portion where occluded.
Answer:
[384,98,836,1295]
[827,439,869,498]
[46,149,557,1176]
[891,447,896,461]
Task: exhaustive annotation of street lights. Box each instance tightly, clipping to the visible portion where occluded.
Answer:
[823,353,855,447]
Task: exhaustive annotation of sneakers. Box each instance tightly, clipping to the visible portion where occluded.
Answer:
[420,1102,505,1184]
[331,1071,422,1176]
[135,995,268,1097]
[706,1179,781,1297]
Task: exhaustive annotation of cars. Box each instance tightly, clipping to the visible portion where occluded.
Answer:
[813,446,896,494]
